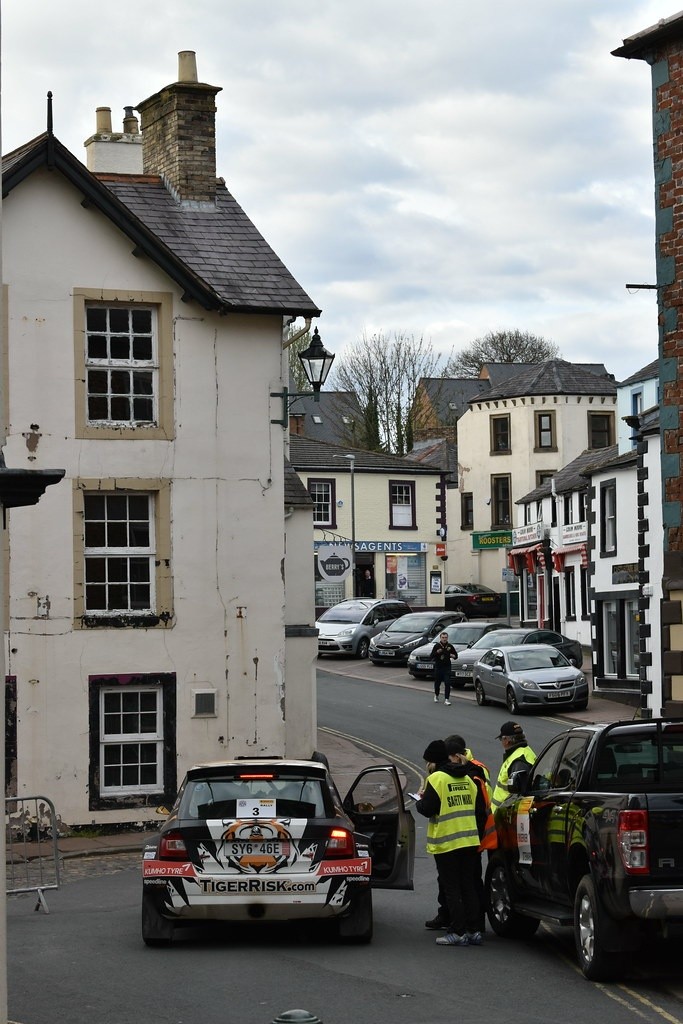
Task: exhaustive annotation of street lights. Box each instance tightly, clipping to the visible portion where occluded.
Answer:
[333,454,357,598]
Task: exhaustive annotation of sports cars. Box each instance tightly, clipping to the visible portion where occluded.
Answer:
[140,751,415,947]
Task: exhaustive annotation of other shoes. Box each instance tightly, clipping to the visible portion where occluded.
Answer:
[434,696,439,702]
[444,700,451,706]
[425,917,447,929]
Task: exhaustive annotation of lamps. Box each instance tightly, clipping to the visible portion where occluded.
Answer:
[270,326,336,428]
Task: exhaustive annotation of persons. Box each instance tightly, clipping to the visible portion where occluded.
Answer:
[490,721,539,815]
[362,569,374,599]
[548,768,605,893]
[430,632,458,706]
[416,734,497,947]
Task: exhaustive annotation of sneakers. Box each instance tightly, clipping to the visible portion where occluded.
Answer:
[436,932,469,946]
[468,931,482,945]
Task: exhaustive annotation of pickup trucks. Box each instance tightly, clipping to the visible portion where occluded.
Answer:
[482,716,683,985]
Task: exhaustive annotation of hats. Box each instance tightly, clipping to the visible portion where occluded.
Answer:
[445,734,466,755]
[423,739,449,763]
[495,722,523,739]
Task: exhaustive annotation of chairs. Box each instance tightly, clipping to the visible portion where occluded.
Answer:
[594,747,617,783]
[666,751,683,780]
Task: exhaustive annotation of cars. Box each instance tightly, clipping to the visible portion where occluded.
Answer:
[444,583,503,617]
[472,643,589,715]
[450,627,584,690]
[368,611,469,667]
[406,622,512,682]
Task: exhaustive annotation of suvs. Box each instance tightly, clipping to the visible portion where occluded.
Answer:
[314,596,413,659]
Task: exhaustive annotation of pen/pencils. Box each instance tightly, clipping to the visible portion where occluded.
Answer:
[418,790,425,795]
